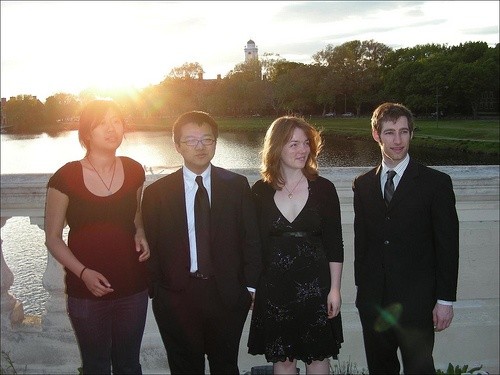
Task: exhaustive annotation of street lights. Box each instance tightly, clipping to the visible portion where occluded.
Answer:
[436,86,449,128]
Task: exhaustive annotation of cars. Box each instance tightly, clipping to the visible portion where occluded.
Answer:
[341,112,353,118]
[324,111,337,118]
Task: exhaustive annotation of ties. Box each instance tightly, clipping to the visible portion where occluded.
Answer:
[384,170,397,209]
[194,176,211,277]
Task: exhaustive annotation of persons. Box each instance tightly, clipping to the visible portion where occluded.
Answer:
[246,115,345,375]
[43,98,150,375]
[352,103,460,375]
[141,110,258,375]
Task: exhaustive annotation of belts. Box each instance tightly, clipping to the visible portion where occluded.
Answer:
[190,272,209,279]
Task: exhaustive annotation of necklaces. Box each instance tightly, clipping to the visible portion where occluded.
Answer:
[282,174,304,199]
[85,156,117,196]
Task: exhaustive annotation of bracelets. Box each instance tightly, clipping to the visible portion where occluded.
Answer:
[80,266,88,280]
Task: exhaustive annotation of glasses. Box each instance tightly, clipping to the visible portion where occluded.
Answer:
[181,139,216,147]
